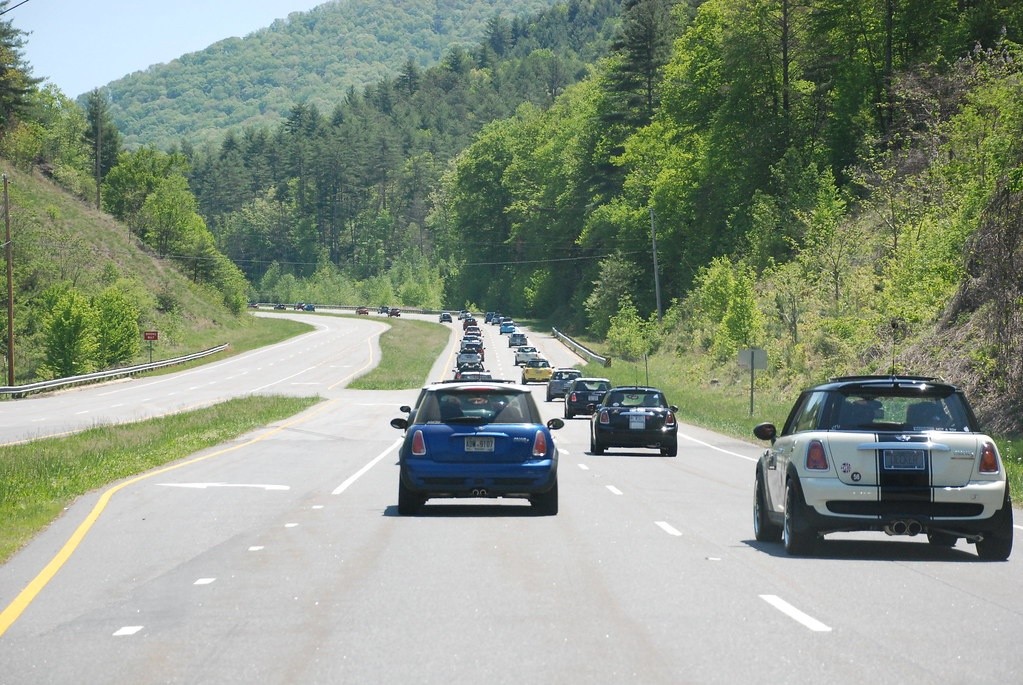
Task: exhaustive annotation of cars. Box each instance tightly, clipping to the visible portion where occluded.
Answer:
[294,302,305,310]
[388,307,401,318]
[752,374,1014,561]
[398,309,680,457]
[390,380,566,517]
[248,303,259,309]
[355,306,369,315]
[377,306,389,314]
[274,305,286,310]
[303,304,315,311]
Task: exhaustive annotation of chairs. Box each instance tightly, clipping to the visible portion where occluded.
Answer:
[569,375,577,380]
[841,405,874,423]
[614,392,624,402]
[530,349,534,352]
[558,373,563,379]
[644,396,657,403]
[597,384,606,391]
[577,383,587,391]
[495,406,522,422]
[542,363,547,367]
[522,349,524,351]
[430,404,464,421]
[907,402,950,426]
